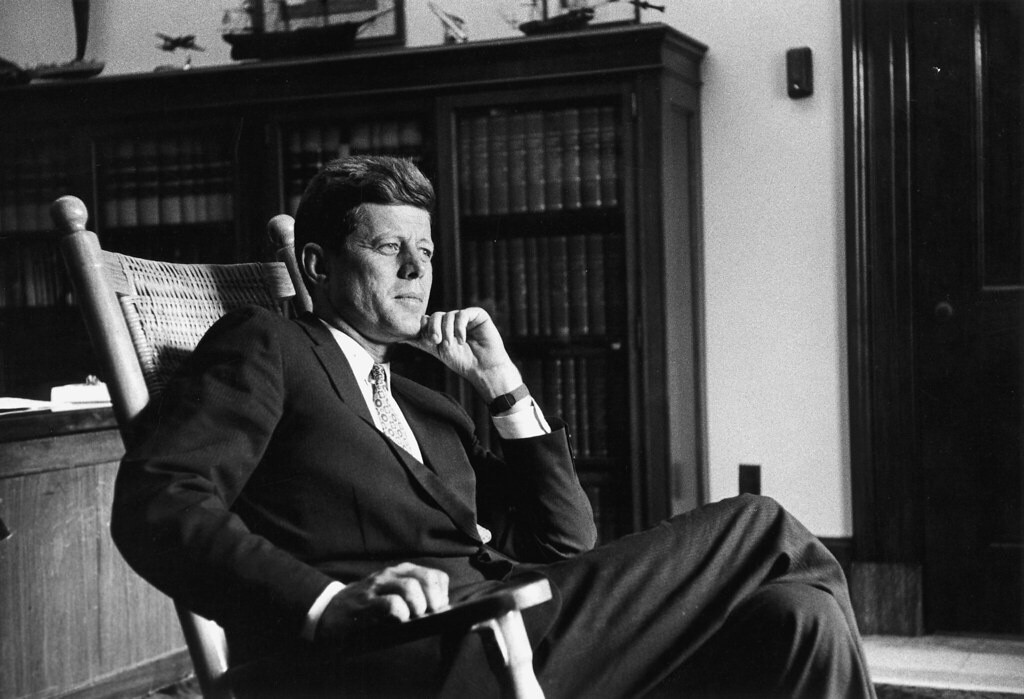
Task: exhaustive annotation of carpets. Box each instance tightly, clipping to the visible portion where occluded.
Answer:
[874,681,1024,699]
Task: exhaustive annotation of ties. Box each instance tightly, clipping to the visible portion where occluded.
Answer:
[366,363,411,456]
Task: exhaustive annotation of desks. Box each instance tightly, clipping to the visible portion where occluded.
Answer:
[1,406,199,698]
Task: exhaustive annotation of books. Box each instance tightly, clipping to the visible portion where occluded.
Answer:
[456,107,629,541]
[282,112,435,222]
[96,121,236,227]
[0,132,98,308]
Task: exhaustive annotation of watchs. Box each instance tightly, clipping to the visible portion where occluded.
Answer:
[487,384,530,417]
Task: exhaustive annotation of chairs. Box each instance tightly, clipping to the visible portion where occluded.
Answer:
[50,190,558,698]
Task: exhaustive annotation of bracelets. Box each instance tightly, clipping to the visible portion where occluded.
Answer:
[109,158,878,699]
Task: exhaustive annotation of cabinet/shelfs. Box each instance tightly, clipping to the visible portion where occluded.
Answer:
[0,18,708,549]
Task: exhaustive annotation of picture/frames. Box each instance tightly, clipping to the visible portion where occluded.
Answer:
[250,0,406,52]
